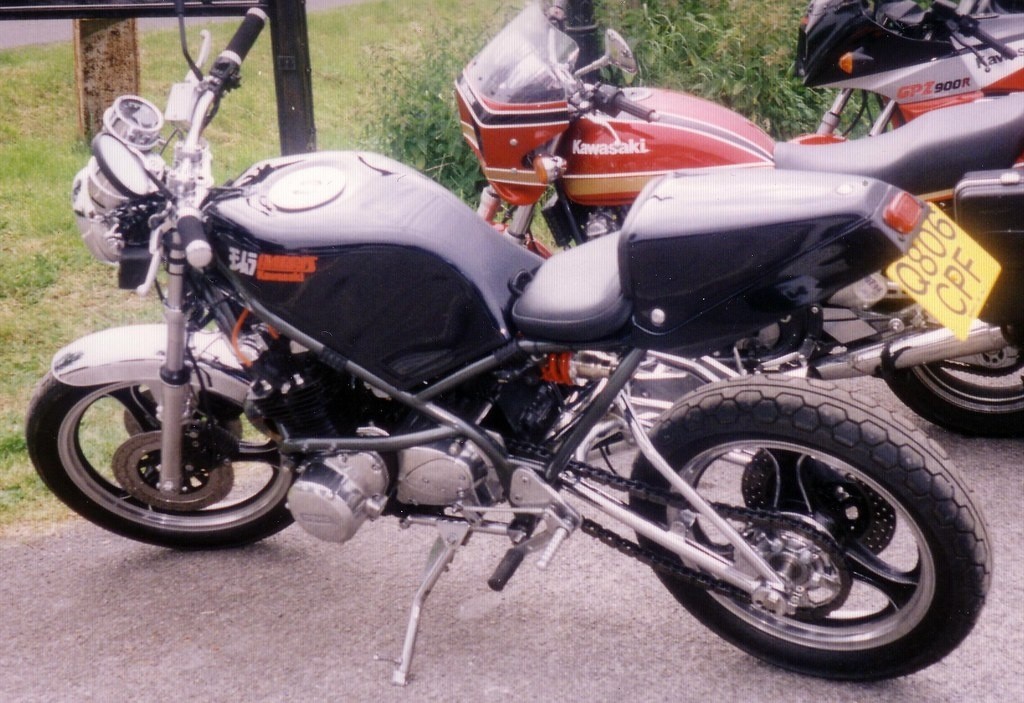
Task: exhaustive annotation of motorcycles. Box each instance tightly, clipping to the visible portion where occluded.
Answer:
[789,0,1024,156]
[449,1,1024,444]
[20,6,1018,686]
